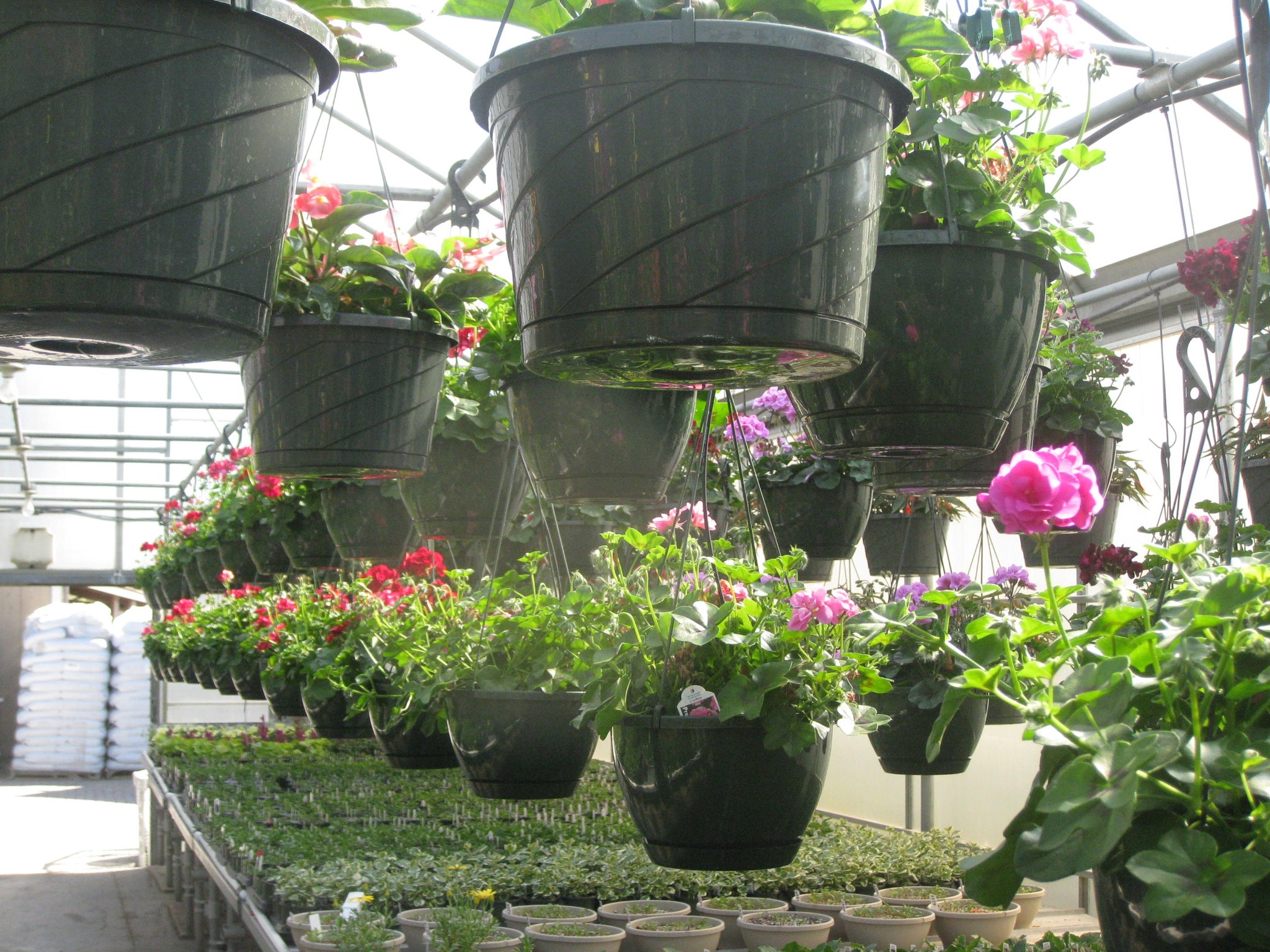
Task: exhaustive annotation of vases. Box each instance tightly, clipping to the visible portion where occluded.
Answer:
[624,915,725,952]
[501,904,597,937]
[597,899,691,930]
[872,886,964,937]
[524,922,626,952]
[300,927,405,952]
[791,892,882,942]
[839,904,936,951]
[958,885,1046,929]
[695,896,790,950]
[422,926,524,952]
[396,907,494,951]
[736,911,835,952]
[0,0,1121,875]
[286,910,386,951]
[927,898,1021,950]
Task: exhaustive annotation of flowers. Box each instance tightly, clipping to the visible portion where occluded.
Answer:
[141,0,1270,952]
